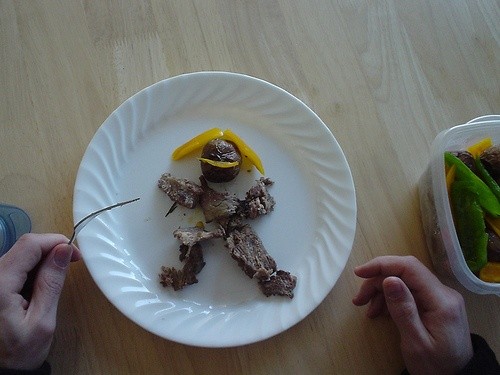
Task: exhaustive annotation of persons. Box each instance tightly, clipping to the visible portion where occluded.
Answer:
[0,231,500,375]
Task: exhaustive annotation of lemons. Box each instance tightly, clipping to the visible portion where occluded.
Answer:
[478,262,500,283]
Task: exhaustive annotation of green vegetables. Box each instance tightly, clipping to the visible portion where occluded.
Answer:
[440,153,500,273]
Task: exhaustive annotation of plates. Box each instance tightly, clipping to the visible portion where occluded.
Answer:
[71,70,358,349]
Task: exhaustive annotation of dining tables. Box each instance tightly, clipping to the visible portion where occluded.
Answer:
[0,0,500,375]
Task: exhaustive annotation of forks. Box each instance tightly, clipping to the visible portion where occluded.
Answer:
[67,197,141,244]
